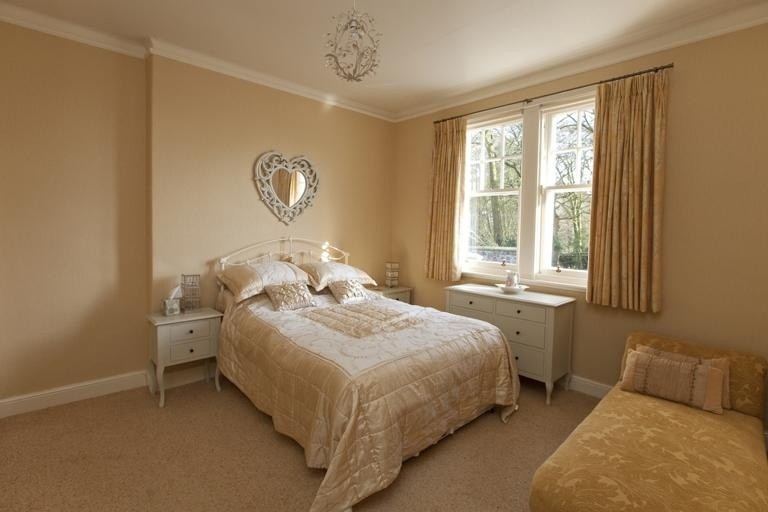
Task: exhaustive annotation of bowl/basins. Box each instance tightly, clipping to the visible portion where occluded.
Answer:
[494,283,529,294]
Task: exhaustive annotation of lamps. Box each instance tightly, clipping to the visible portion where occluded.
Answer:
[322,1,383,83]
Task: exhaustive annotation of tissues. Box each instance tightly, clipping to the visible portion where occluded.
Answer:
[160,283,184,316]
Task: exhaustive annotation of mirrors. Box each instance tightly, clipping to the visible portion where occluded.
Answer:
[254,150,320,226]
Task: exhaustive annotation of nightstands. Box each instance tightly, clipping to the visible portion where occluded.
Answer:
[145,307,225,408]
[370,284,413,304]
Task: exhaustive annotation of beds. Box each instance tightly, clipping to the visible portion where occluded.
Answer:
[212,237,522,511]
[527,332,767,512]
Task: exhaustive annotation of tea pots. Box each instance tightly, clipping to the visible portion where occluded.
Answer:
[503,269,520,288]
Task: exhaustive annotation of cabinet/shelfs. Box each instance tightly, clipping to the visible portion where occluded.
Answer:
[444,282,577,406]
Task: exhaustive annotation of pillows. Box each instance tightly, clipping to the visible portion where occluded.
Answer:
[264,280,318,312]
[216,261,319,304]
[619,333,766,420]
[634,342,734,410]
[298,260,378,293]
[620,347,724,415]
[328,278,377,305]
